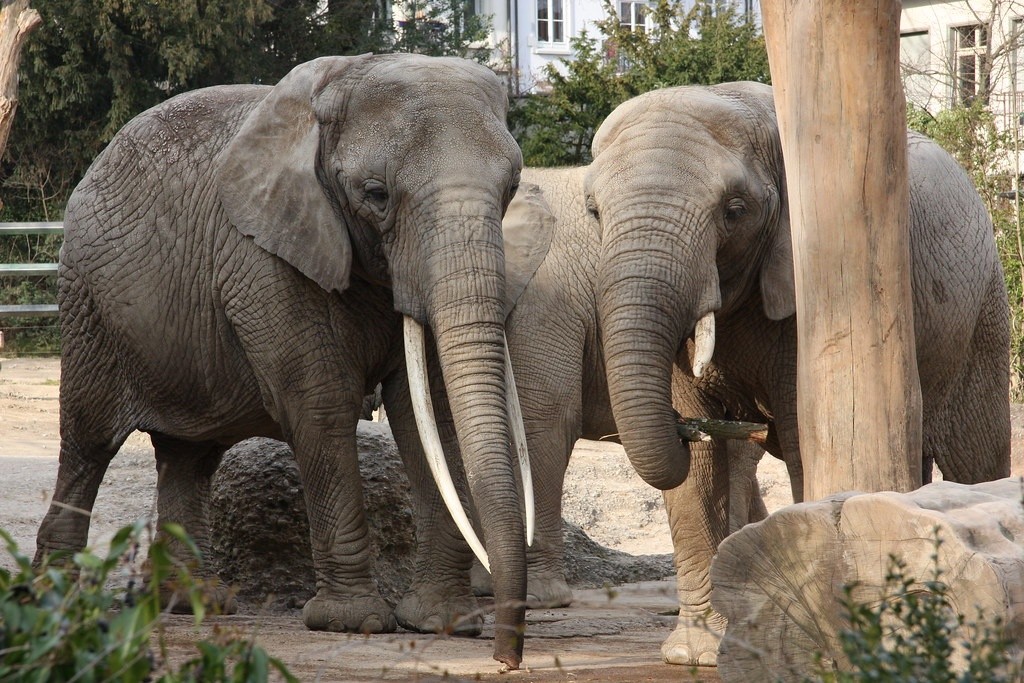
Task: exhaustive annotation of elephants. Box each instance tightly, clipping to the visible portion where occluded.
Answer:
[35,53,1013,667]
